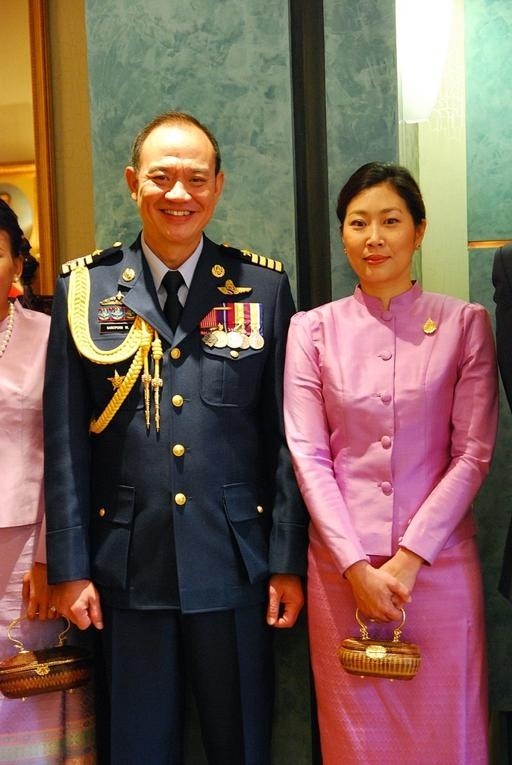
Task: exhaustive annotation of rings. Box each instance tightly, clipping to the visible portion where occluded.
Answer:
[369,618,376,622]
[49,607,56,613]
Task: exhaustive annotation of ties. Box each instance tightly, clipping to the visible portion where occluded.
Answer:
[162,271,185,337]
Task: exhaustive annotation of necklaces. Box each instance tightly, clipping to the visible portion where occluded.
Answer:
[0,300,17,358]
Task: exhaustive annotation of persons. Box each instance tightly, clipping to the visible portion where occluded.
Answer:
[0,193,99,764]
[281,156,497,765]
[42,111,311,763]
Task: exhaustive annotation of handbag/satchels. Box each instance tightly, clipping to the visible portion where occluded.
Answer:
[0,611,92,700]
[337,604,422,681]
